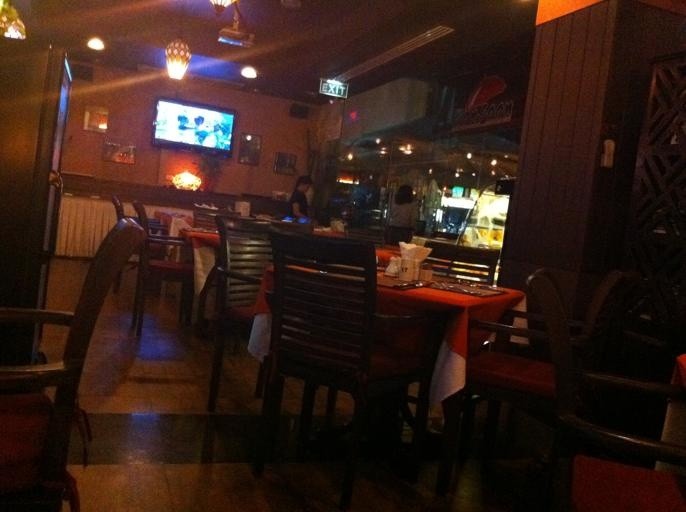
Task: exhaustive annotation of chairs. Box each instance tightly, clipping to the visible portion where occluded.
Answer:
[0,217,144,512]
[207,198,686,512]
[108,192,379,337]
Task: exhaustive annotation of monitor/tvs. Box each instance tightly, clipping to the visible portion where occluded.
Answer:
[151,95,236,157]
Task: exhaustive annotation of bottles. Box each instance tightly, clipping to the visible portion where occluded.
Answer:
[384,255,403,278]
[418,262,434,281]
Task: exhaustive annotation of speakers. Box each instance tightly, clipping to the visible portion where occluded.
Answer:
[71,65,91,79]
[291,103,309,117]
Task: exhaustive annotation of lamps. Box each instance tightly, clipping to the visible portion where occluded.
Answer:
[164,33,193,82]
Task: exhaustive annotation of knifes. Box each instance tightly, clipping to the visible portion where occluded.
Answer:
[393,281,424,288]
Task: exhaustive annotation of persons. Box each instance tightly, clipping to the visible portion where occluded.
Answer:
[288,175,312,217]
[384,185,417,246]
